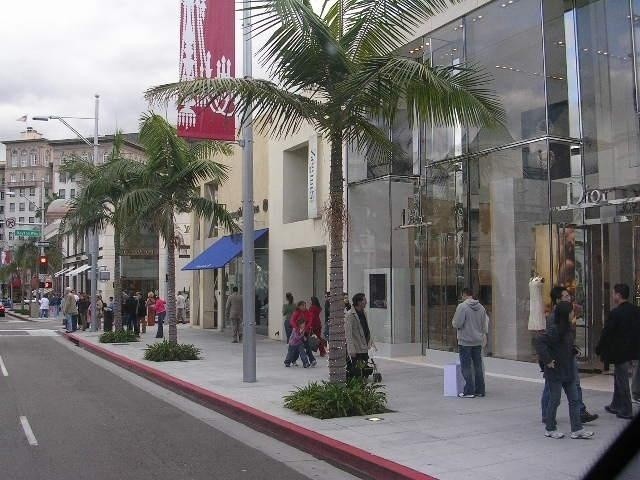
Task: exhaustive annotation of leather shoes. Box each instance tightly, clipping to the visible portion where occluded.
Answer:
[580,411,598,423]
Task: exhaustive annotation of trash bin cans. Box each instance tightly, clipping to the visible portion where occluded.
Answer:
[103,307,114,332]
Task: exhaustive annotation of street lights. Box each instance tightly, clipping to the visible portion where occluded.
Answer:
[31,94,100,334]
[5,178,46,299]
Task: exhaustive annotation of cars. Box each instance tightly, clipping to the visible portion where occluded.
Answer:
[0,302,5,317]
[21,297,37,305]
[1,299,11,308]
[49,296,61,306]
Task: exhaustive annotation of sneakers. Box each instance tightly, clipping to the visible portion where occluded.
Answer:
[545,430,565,439]
[283,360,317,368]
[458,392,475,398]
[476,394,485,397]
[571,428,596,439]
[605,397,640,419]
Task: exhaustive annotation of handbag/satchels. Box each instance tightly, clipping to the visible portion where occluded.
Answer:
[307,327,321,352]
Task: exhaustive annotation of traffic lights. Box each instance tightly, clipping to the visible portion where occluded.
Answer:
[39,256,47,275]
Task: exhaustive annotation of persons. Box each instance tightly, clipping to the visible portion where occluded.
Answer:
[39,286,166,339]
[533,301,595,439]
[595,285,640,418]
[344,293,372,382]
[452,287,487,397]
[343,293,351,311]
[226,287,243,343]
[528,272,546,332]
[175,291,187,326]
[542,286,599,425]
[283,291,330,367]
